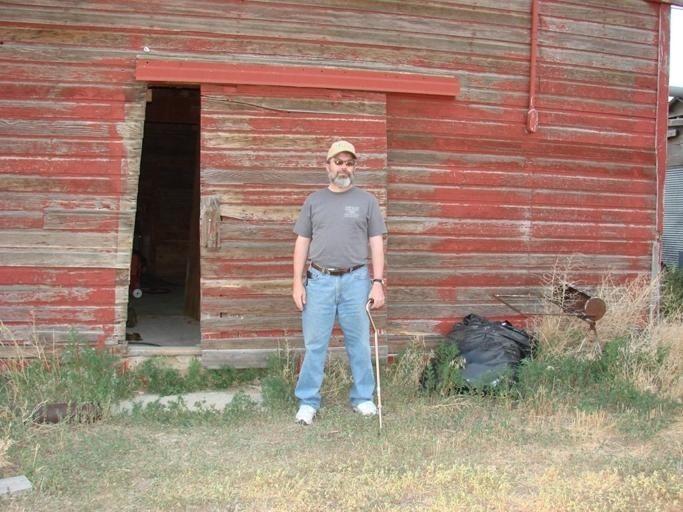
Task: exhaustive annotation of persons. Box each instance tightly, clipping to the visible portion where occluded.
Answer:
[292,141,387,426]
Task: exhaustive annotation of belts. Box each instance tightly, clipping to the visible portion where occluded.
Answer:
[311,263,364,276]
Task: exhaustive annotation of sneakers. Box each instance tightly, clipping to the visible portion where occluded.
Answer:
[294,406,316,426]
[355,399,378,415]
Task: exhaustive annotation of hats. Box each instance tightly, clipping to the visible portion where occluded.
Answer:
[327,141,357,161]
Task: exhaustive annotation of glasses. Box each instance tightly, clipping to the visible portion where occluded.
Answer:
[334,157,355,166]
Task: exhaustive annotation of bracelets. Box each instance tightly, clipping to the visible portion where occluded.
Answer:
[370,279,384,285]
